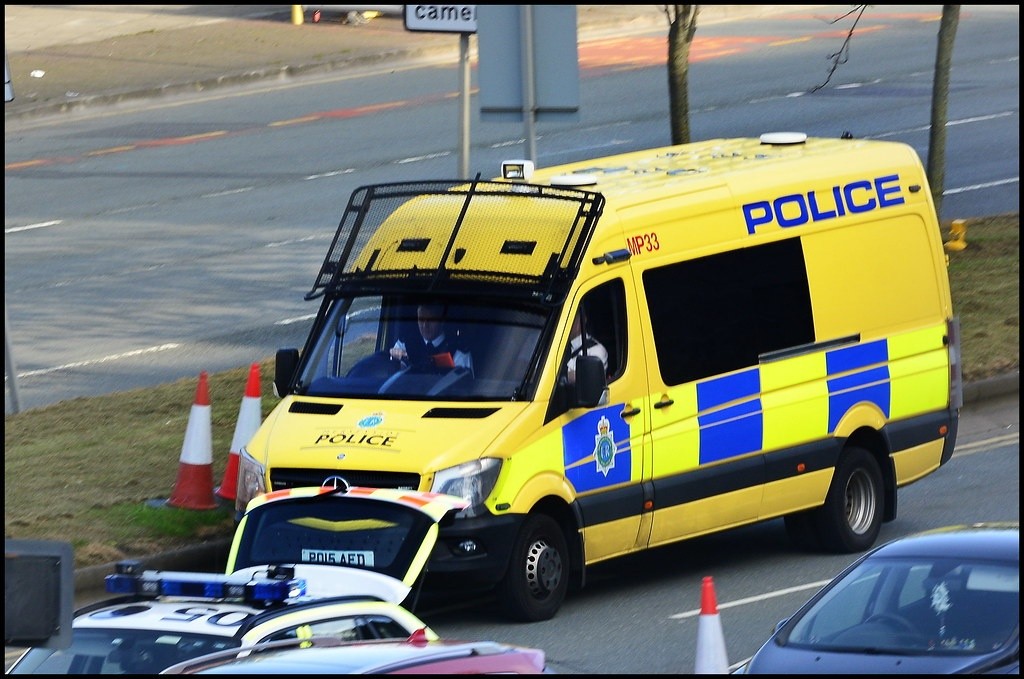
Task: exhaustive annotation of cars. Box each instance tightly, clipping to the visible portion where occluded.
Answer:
[6,486,472,674]
[159,629,581,674]
[731,523,1020,675]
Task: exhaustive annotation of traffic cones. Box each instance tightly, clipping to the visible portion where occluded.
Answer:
[215,362,262,500]
[145,371,220,512]
[695,576,729,675]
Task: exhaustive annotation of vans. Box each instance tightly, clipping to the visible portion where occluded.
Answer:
[233,131,963,622]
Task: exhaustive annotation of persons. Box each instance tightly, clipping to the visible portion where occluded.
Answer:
[553,307,609,399]
[401,305,467,375]
